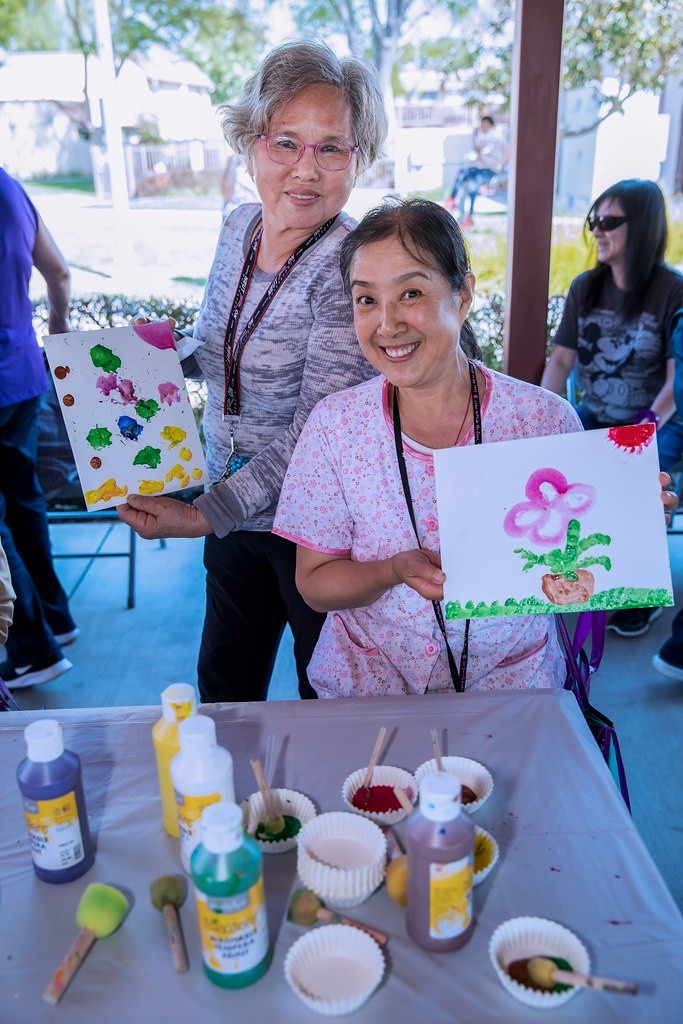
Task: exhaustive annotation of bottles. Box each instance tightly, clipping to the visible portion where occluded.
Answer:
[170,715,236,876]
[150,684,200,840]
[189,801,273,990]
[404,772,476,952]
[16,718,95,886]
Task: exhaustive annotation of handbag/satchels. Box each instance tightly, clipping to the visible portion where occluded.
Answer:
[576,647,614,768]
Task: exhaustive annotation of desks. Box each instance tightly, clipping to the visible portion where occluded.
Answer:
[0,687,683,1024]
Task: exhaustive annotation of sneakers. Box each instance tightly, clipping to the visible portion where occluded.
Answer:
[652,635,683,684]
[444,196,455,211]
[0,648,75,690]
[460,215,473,228]
[52,621,80,647]
[605,607,664,638]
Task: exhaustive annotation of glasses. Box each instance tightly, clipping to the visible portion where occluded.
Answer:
[587,214,628,232]
[257,132,360,172]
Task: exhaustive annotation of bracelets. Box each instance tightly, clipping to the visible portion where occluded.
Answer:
[645,410,661,430]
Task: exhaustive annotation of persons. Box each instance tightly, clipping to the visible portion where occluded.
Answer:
[222,152,262,225]
[271,198,678,700]
[0,169,79,689]
[442,116,505,229]
[541,178,683,680]
[117,41,389,704]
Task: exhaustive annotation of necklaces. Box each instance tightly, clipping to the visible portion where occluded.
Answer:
[453,387,472,446]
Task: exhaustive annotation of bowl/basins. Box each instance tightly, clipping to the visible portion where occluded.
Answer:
[414,754,494,813]
[472,824,501,888]
[295,813,388,909]
[341,765,417,825]
[240,788,318,855]
[283,923,385,1017]
[487,915,592,1009]
